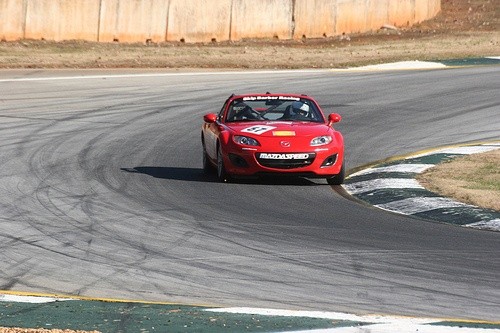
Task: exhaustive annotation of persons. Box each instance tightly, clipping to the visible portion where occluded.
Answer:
[291,102,309,117]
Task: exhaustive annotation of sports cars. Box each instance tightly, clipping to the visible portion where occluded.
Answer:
[201,93,345,186]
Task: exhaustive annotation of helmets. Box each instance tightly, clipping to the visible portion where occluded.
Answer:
[290,102,309,118]
[230,103,252,119]
